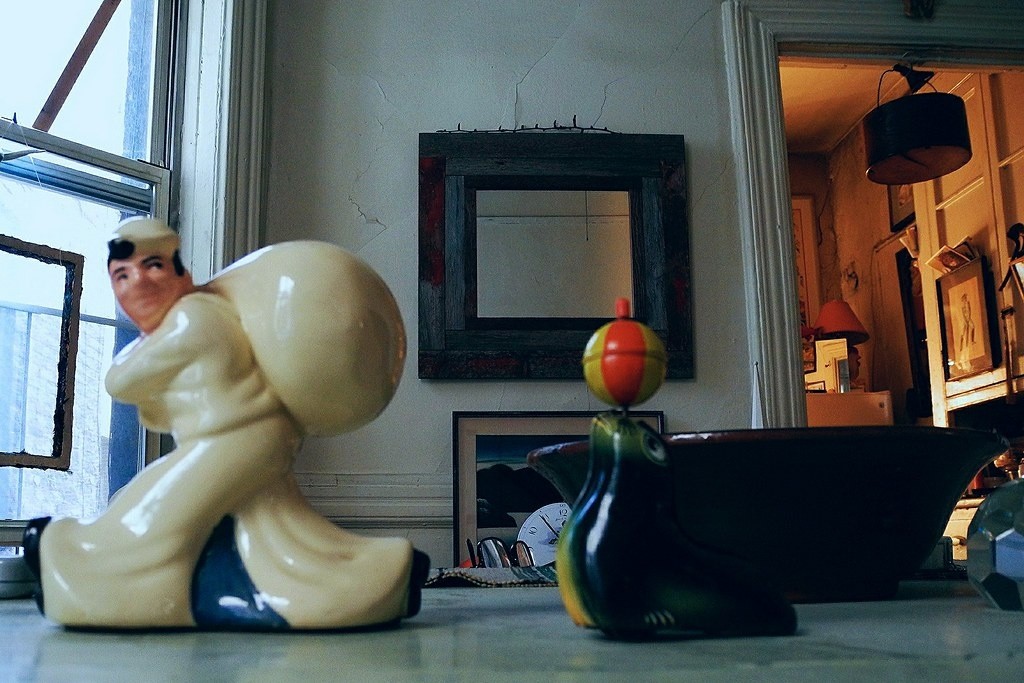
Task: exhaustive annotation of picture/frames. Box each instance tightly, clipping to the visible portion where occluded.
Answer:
[935,255,997,381]
[451,411,665,568]
[887,184,916,232]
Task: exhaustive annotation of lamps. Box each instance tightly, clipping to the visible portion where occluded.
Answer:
[812,301,870,358]
[893,61,934,93]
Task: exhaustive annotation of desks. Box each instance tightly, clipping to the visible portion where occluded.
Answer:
[661,426,1010,605]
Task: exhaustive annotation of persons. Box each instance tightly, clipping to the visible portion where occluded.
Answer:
[22,217,431,632]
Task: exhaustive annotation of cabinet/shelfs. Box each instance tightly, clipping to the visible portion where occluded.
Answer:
[911,73,1024,562]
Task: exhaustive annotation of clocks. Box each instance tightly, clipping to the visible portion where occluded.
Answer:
[516,503,572,567]
